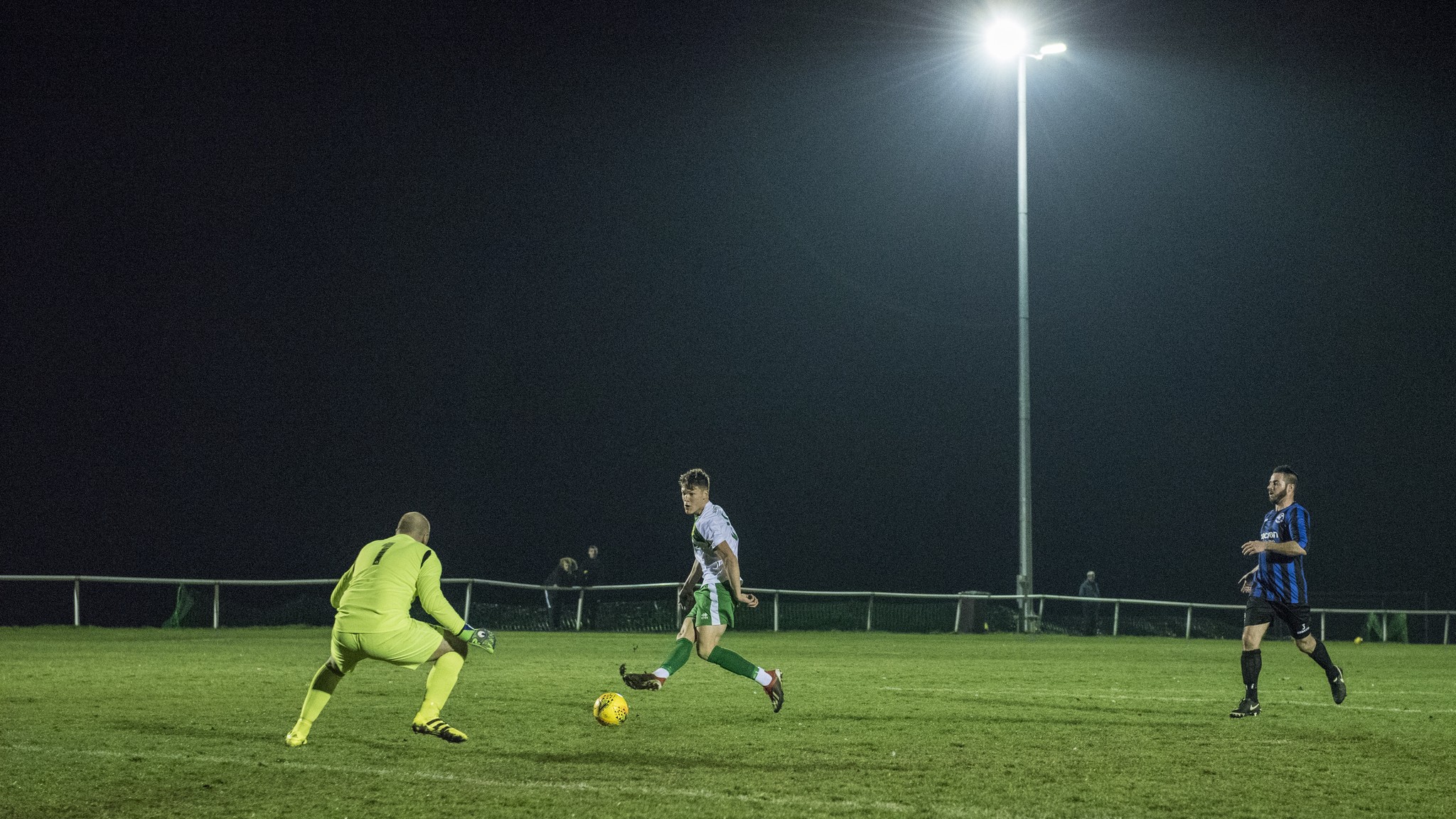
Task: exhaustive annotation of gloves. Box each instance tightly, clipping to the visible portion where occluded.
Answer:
[457,623,496,654]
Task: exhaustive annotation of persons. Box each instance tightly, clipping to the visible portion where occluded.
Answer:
[622,468,784,713]
[1229,465,1346,717]
[577,545,606,630]
[1078,571,1100,634]
[285,512,496,746]
[548,558,578,631]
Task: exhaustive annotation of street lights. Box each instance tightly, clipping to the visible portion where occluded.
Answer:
[984,15,1034,628]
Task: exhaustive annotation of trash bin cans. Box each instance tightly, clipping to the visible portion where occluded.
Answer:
[954,591,990,633]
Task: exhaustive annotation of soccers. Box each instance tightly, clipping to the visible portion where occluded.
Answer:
[593,693,629,728]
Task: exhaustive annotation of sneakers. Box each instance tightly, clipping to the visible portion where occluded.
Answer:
[623,671,666,692]
[412,712,467,744]
[286,730,307,747]
[1326,665,1347,705]
[763,669,784,713]
[1230,697,1261,718]
